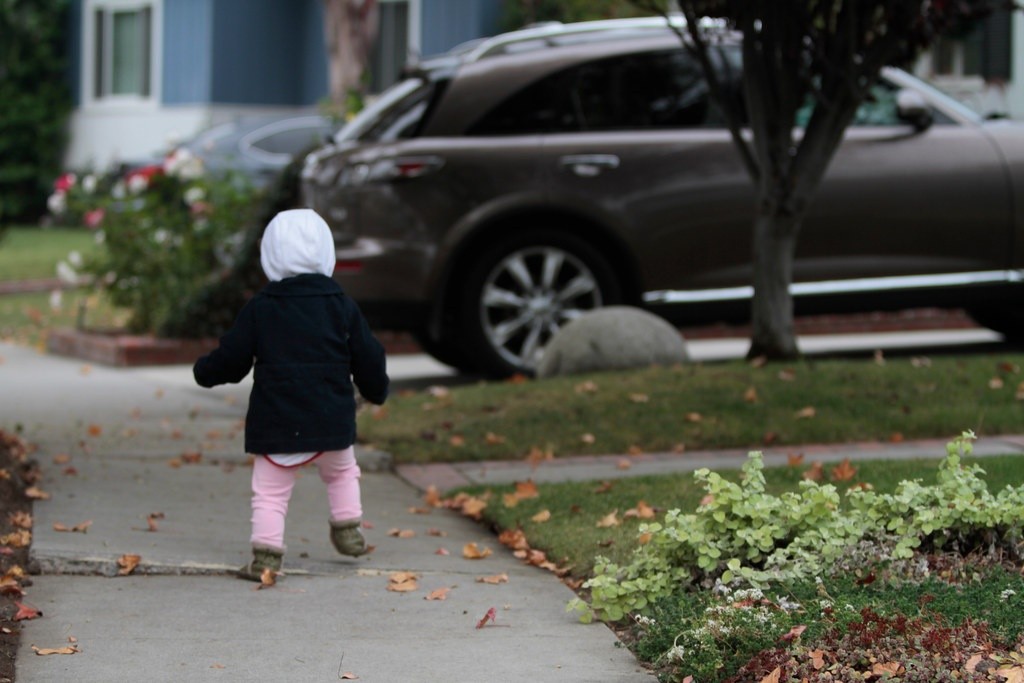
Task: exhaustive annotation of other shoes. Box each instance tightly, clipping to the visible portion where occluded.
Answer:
[330,518,372,555]
[238,551,281,584]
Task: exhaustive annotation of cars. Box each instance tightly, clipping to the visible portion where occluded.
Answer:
[116,112,332,203]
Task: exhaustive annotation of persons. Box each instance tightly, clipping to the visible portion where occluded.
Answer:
[193,209,390,583]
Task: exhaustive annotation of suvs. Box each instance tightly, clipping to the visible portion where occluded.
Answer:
[303,16,1024,378]
